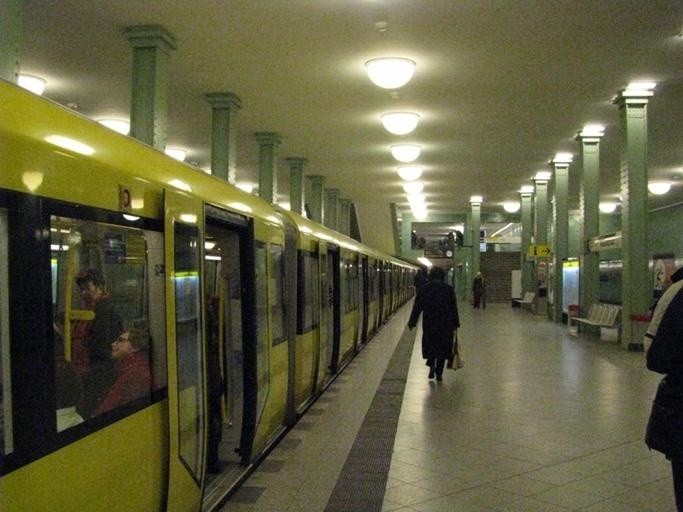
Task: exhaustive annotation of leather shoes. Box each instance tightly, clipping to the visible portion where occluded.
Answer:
[428,373,443,381]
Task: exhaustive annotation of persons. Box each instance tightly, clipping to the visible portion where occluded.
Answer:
[471,272,483,308]
[643,266,683,512]
[411,228,463,295]
[96,324,152,415]
[75,268,126,421]
[408,266,460,380]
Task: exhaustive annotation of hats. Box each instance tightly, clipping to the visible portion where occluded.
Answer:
[429,266,445,281]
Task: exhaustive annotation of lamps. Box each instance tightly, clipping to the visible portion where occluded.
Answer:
[364,56,424,164]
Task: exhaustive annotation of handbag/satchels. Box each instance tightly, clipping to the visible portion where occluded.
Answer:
[446,344,465,370]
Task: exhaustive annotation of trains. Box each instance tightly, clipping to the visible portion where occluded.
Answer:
[0,77,428,512]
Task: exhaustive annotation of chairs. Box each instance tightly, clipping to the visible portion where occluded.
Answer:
[516,291,535,313]
[570,302,621,341]
